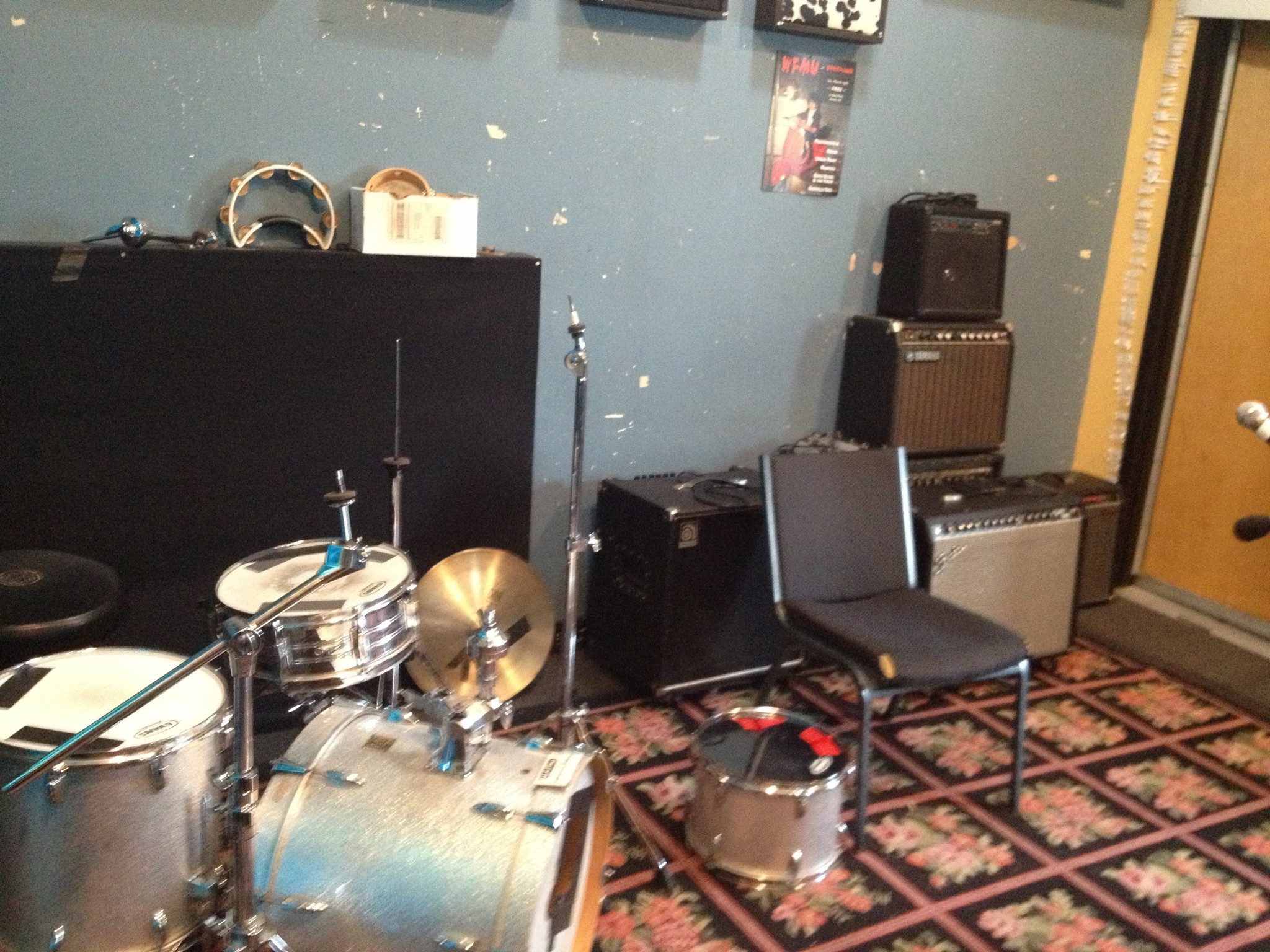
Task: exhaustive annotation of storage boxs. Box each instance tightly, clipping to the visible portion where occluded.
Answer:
[348,187,481,260]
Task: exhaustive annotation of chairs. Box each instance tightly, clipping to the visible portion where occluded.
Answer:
[759,445,1034,830]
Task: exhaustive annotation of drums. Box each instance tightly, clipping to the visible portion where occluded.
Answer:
[679,705,857,892]
[249,693,614,952]
[0,645,237,952]
[208,535,419,695]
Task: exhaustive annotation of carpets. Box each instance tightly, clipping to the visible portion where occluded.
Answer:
[487,634,1270,950]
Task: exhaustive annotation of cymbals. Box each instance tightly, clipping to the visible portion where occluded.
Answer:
[405,548,555,708]
[218,160,339,250]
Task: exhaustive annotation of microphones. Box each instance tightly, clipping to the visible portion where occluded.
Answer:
[1235,399,1270,447]
[1234,514,1269,540]
